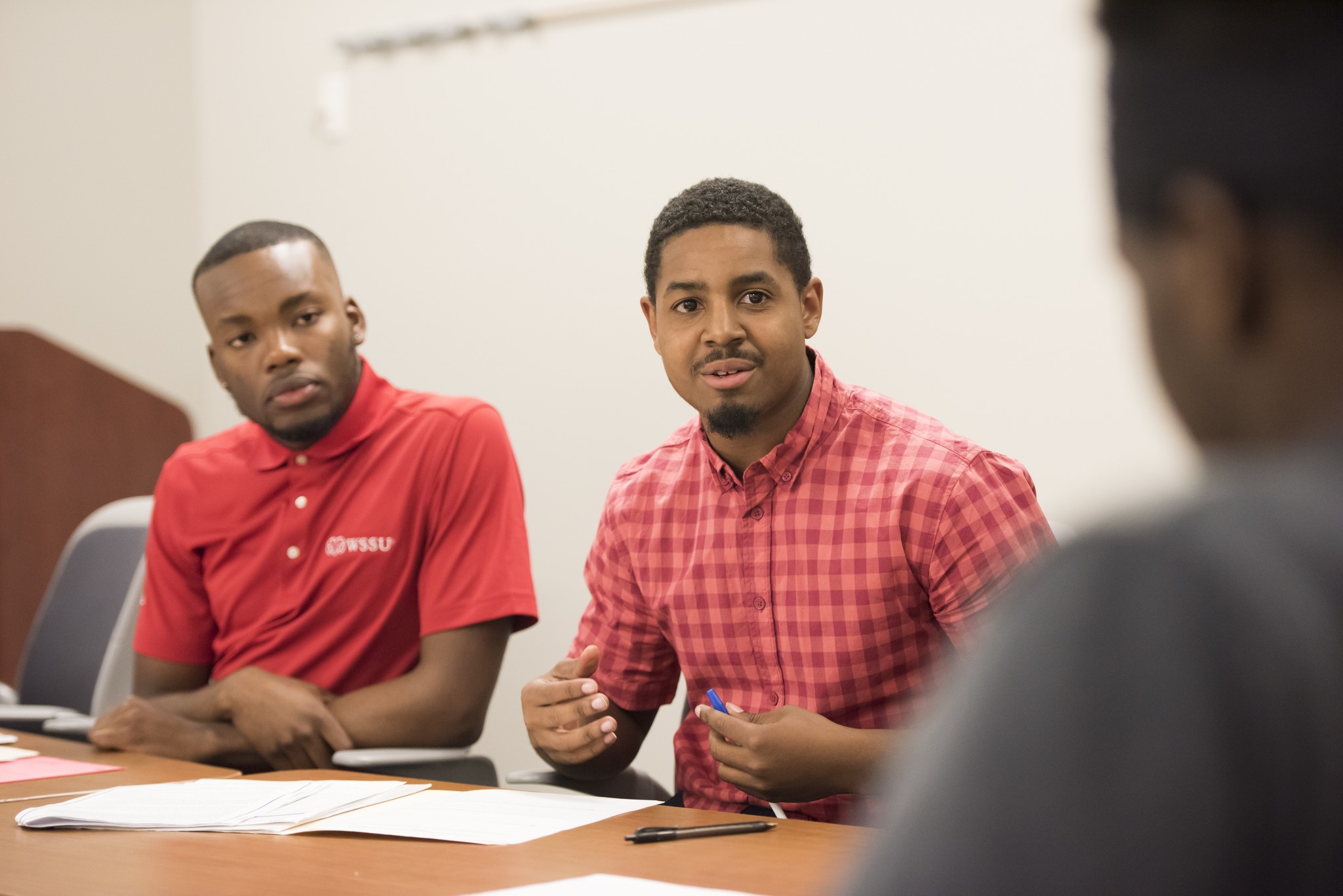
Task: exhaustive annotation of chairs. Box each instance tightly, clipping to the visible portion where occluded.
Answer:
[14,494,500,789]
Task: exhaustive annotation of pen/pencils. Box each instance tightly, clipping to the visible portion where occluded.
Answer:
[623,820,777,844]
[706,688,788,819]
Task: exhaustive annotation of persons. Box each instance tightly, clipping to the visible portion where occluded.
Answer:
[521,174,1057,827]
[86,221,541,771]
[823,0,1343,896]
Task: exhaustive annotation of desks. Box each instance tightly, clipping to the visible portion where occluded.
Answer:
[0,728,874,896]
